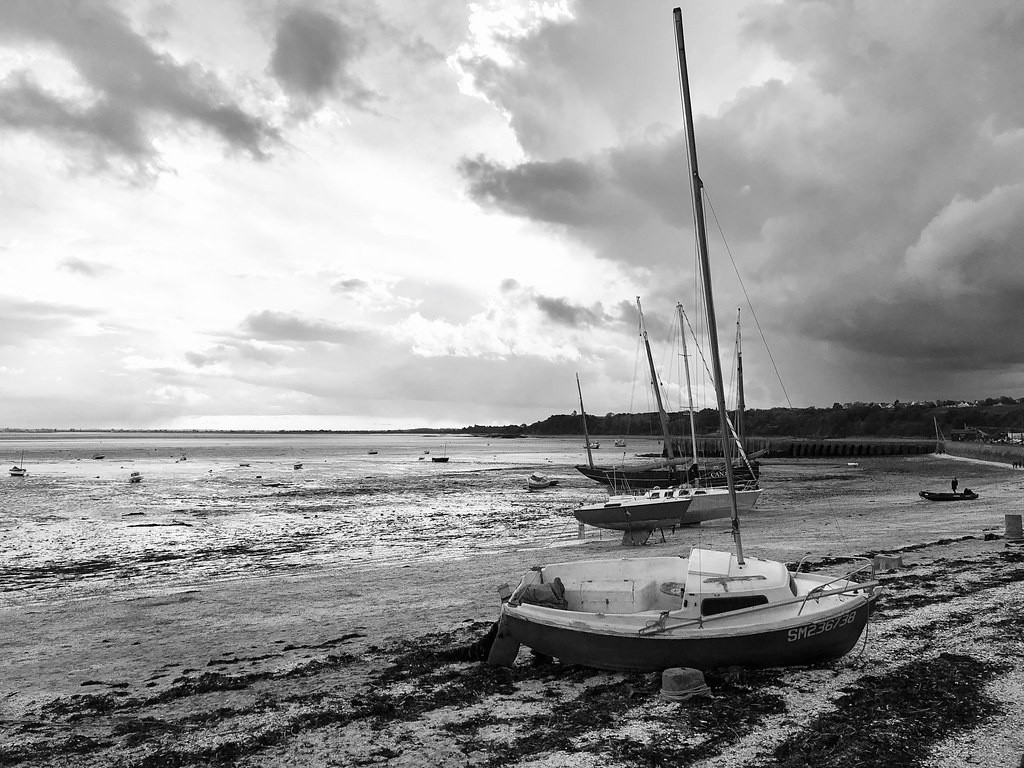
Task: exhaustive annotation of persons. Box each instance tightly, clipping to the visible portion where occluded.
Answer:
[1012,460,1024,468]
[952,478,958,493]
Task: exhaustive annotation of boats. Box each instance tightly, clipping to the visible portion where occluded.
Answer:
[573,492,693,549]
[499,6,879,675]
[918,488,979,500]
[583,442,599,449]
[432,456,450,463]
[368,450,378,455]
[614,439,626,447]
[94,454,104,459]
[525,472,558,489]
[574,294,765,525]
[9,466,27,476]
